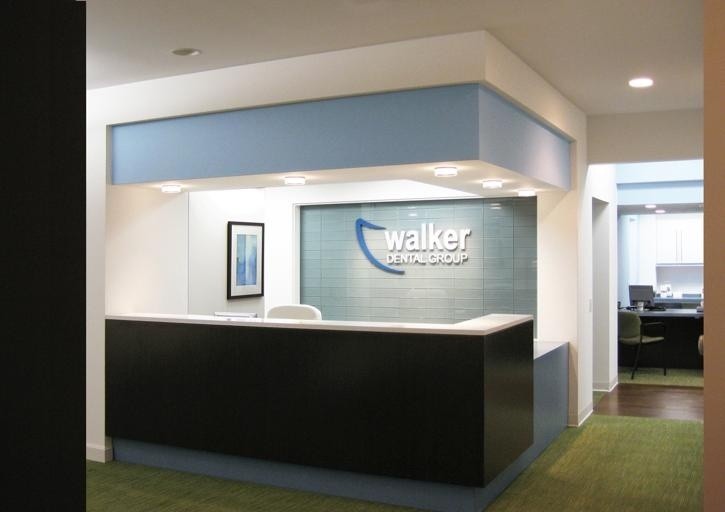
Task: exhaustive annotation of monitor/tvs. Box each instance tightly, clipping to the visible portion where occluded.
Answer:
[214,312,257,318]
[628,284,654,312]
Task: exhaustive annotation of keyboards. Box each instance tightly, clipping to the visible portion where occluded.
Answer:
[627,305,667,311]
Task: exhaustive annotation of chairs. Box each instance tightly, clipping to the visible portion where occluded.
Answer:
[266,304,323,320]
[618,310,667,380]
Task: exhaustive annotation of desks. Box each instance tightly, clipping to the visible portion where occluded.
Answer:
[618,308,704,369]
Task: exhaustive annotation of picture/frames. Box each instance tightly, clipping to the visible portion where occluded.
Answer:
[227,221,265,299]
